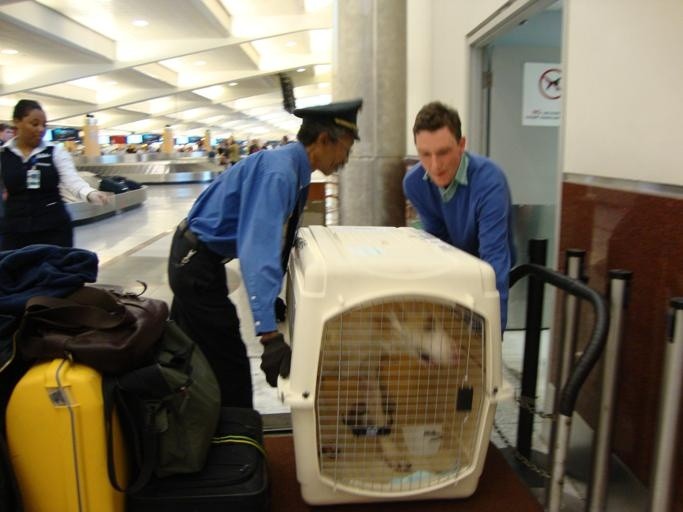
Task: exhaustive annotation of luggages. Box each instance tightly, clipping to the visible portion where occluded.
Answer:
[5,357,127,511]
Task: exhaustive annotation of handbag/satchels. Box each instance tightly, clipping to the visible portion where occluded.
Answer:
[0,244,223,494]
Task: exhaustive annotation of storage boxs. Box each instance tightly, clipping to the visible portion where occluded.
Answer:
[285,225,503,504]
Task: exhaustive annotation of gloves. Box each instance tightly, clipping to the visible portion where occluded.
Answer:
[276,297,286,323]
[259,333,292,388]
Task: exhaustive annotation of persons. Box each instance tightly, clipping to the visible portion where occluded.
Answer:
[168,101,362,408]
[177,135,288,166]
[402,103,517,340]
[0,100,110,251]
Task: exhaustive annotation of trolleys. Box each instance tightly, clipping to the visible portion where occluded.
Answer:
[260,264,609,512]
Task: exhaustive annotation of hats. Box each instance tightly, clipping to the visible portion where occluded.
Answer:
[292,98,363,141]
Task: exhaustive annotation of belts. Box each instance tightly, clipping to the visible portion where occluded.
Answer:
[178,222,213,260]
